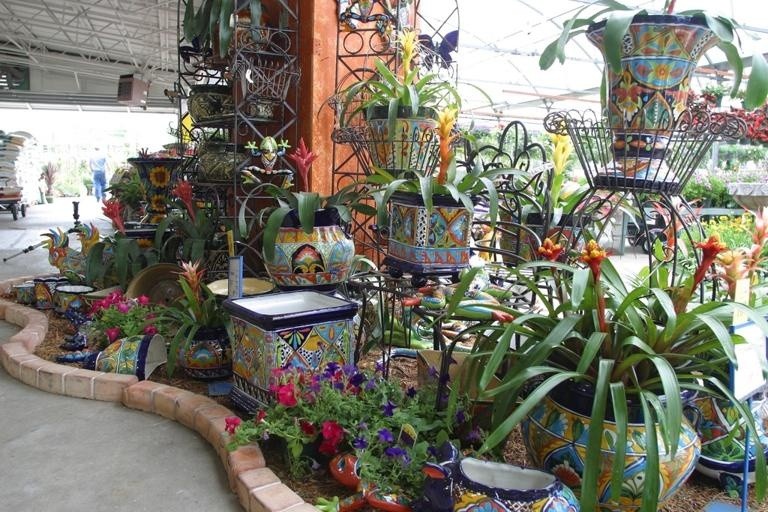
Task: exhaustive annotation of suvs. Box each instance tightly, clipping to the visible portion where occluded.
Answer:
[470,205,614,252]
[626,207,755,255]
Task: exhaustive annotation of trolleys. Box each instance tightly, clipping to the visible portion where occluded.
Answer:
[0,185,27,219]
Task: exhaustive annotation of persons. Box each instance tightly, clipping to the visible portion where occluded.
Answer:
[90,145,111,202]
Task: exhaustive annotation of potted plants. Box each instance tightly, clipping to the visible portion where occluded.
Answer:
[13,0,768,512]
